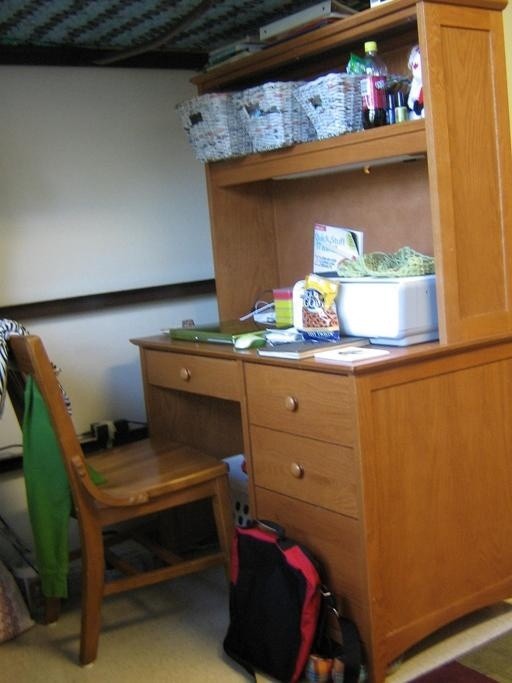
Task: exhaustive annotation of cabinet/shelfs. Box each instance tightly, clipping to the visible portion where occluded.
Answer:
[190,1,512,344]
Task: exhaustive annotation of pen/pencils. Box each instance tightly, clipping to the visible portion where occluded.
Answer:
[239,301,275,321]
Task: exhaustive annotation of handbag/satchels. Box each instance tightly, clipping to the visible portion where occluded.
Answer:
[0,515,37,644]
[223,521,322,683]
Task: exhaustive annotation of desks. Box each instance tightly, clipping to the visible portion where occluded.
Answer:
[128,321,512,682]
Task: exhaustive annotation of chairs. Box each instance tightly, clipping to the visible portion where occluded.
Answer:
[2,335,235,666]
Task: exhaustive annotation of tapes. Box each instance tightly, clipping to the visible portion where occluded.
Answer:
[251,300,267,311]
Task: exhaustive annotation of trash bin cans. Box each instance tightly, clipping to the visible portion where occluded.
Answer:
[225,456,247,530]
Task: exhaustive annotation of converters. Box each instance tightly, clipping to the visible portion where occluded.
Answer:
[90,418,130,440]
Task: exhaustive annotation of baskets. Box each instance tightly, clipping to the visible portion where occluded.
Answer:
[173,73,362,164]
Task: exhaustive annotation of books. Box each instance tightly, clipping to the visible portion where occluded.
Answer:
[314,345,392,367]
[255,335,371,360]
[204,0,359,72]
[311,221,364,272]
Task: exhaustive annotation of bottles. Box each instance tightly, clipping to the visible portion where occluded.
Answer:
[359,39,389,130]
